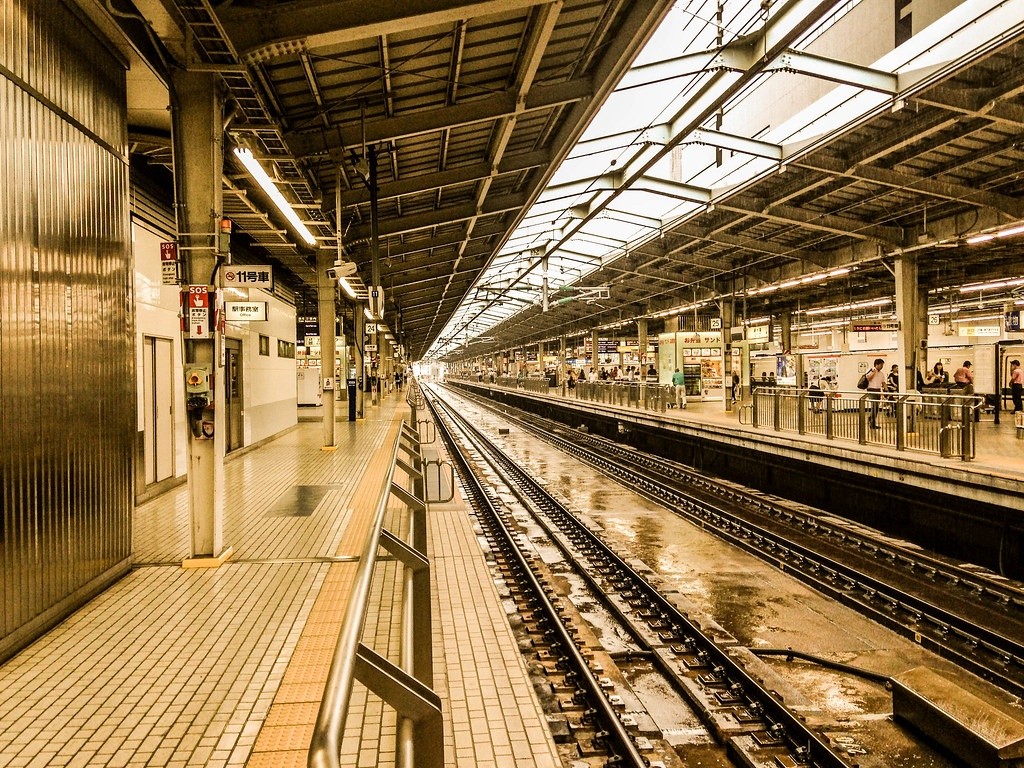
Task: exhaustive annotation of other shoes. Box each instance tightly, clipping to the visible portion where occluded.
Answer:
[870,424,882,430]
[813,409,822,414]
[679,402,687,409]
[1010,409,1022,414]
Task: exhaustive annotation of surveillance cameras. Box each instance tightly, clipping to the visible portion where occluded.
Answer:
[326,262,357,280]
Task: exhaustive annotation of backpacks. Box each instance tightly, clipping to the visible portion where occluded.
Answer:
[857,369,873,390]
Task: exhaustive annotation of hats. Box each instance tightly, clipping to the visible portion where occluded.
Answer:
[963,360,973,368]
[1010,360,1020,367]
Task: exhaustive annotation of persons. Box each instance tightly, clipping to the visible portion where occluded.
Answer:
[808,376,832,414]
[567,365,640,402]
[917,370,926,390]
[326,379,330,386]
[785,358,795,377]
[1009,359,1023,415]
[732,370,737,404]
[477,368,556,388]
[672,368,687,409]
[882,364,898,412]
[866,359,889,429]
[707,368,715,377]
[953,361,973,387]
[927,362,945,388]
[647,364,658,375]
[387,371,408,394]
